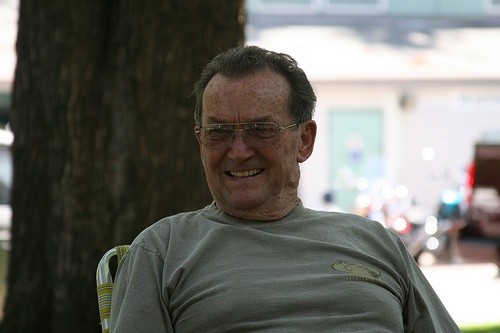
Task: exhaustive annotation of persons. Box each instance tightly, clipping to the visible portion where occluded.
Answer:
[100,43,458,333]
[319,188,347,212]
[353,179,493,264]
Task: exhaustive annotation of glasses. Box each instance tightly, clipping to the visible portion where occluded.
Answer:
[196,117,298,154]
